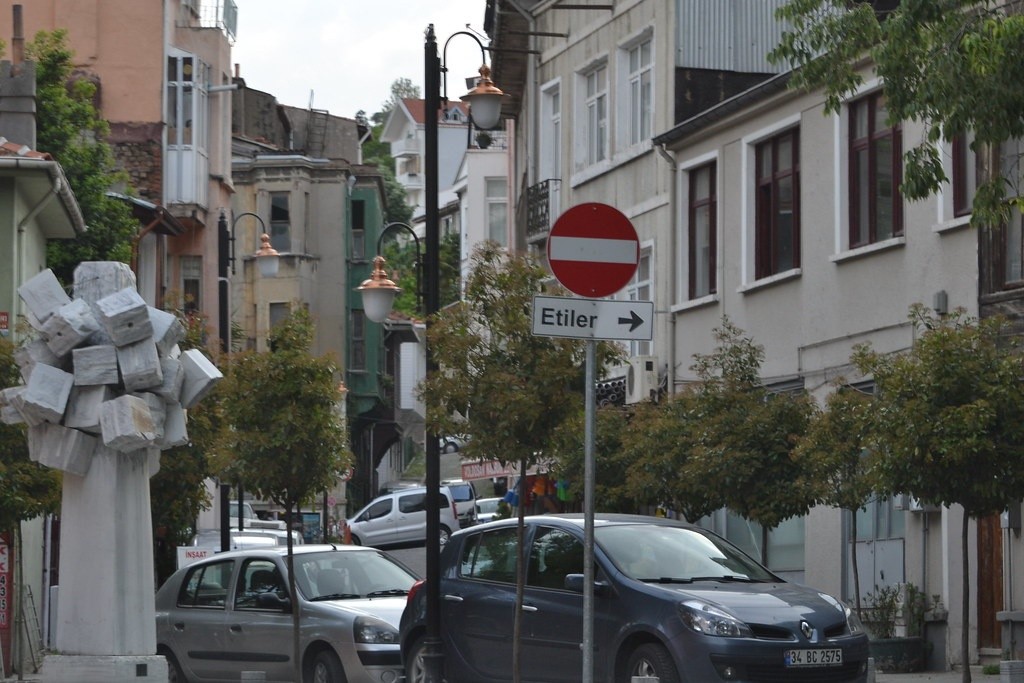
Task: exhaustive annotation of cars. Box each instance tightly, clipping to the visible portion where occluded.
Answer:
[441,479,478,528]
[475,496,510,524]
[398,514,869,683]
[156,545,424,683]
[229,500,304,549]
[348,488,461,548]
[439,433,472,454]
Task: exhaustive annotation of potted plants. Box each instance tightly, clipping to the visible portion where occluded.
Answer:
[848,570,932,673]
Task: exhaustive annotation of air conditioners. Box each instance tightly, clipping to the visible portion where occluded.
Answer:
[626,355,659,404]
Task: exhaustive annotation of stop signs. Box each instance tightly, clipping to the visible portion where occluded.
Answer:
[549,203,641,299]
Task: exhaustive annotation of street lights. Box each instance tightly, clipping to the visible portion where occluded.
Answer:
[218,212,282,587]
[357,21,512,683]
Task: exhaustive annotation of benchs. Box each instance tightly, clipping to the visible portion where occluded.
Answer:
[186,582,254,607]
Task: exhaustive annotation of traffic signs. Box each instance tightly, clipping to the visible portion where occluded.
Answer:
[531,295,655,342]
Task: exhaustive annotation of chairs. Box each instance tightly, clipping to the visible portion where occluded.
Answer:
[541,539,582,589]
[250,570,280,609]
[317,570,347,595]
